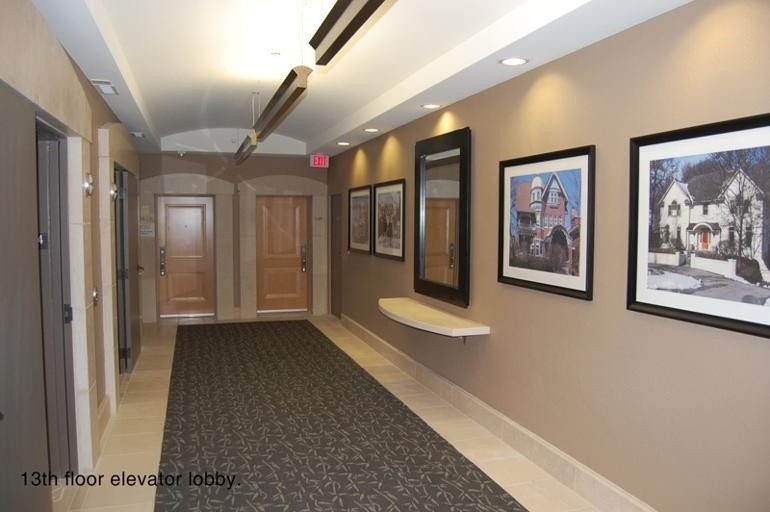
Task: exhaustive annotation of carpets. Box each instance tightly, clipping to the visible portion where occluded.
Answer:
[154,319,526,511]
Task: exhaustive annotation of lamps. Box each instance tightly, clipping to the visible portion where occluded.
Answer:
[231,1,397,168]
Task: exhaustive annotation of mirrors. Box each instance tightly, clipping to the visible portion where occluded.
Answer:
[413,127,472,308]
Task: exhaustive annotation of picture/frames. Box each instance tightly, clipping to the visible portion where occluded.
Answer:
[624,111,770,337]
[498,142,597,304]
[347,177,406,261]
